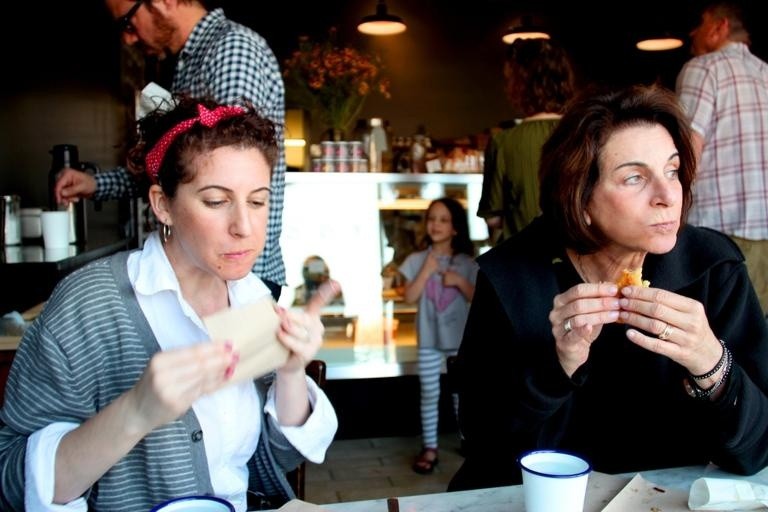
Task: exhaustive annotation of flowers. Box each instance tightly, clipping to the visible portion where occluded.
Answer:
[287,38,391,141]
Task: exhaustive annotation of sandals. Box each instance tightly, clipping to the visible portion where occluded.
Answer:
[412,448,438,473]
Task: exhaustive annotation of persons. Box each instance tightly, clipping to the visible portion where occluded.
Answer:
[478,38,576,240]
[446,81,767,490]
[54,1,287,304]
[0,99,339,511]
[673,0,767,319]
[397,198,480,474]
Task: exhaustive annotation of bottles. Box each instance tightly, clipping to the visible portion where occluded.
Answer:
[0,194,22,245]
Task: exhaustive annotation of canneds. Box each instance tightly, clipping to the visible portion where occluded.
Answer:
[313,139,369,171]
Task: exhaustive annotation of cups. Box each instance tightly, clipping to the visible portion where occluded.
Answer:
[41,209,70,252]
[19,207,43,239]
[150,495,233,511]
[515,449,589,511]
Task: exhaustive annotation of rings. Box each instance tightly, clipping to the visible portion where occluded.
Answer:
[564,318,573,332]
[658,322,672,339]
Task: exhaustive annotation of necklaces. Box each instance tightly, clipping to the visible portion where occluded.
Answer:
[683,339,732,400]
[576,253,592,283]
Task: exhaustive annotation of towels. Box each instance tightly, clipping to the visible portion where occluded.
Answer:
[685,474,766,512]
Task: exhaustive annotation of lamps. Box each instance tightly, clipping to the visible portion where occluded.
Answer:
[354,0,411,38]
[634,33,682,53]
[501,20,550,47]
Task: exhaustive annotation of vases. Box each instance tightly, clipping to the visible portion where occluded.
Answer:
[303,141,372,172]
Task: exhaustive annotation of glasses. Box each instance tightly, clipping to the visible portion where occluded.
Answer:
[118,0,143,30]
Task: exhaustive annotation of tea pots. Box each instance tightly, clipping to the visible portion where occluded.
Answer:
[45,143,102,248]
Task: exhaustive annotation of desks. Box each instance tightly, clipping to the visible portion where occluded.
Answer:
[1,216,138,315]
